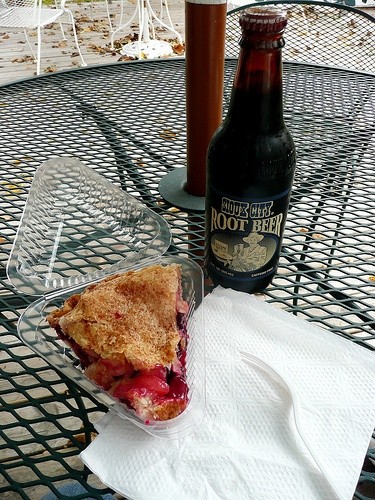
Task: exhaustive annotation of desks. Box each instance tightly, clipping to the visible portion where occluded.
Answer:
[106,0,184,61]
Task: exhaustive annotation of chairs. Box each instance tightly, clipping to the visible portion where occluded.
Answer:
[227,0,313,50]
[0,0,88,76]
[226,0,375,317]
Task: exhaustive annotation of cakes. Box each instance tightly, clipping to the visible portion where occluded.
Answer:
[45,263,190,420]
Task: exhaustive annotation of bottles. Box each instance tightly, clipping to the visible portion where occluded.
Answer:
[206,6,298,296]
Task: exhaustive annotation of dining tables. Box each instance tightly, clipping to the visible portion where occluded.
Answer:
[0,54,375,500]
